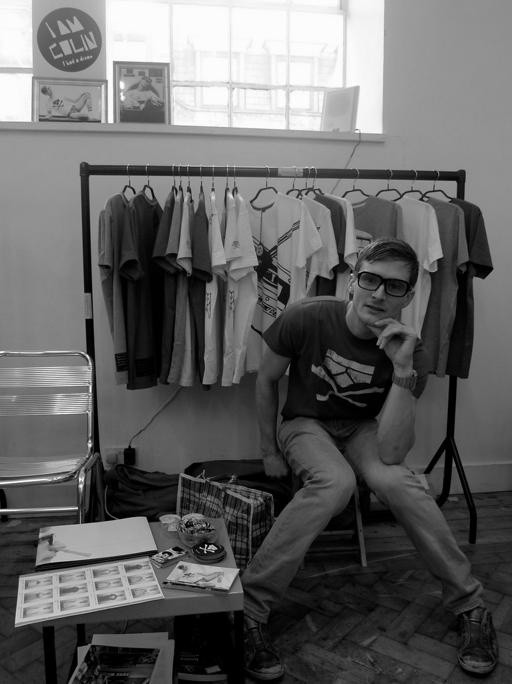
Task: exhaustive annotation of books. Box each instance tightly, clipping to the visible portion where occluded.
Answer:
[71,645,161,684]
[175,649,227,683]
[161,561,240,594]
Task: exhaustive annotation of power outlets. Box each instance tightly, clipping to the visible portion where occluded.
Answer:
[104,446,136,471]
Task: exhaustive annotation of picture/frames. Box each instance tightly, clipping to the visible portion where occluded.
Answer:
[31,76,109,127]
[111,59,171,123]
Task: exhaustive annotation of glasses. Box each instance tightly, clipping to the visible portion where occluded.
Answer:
[357,270,412,297]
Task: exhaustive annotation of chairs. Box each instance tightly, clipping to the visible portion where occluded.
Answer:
[0,350,98,532]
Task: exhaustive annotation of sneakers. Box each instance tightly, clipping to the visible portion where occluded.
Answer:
[456,606,499,674]
[227,613,285,681]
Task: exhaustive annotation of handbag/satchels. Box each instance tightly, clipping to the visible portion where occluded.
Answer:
[101,463,179,522]
[176,469,275,572]
[184,460,267,491]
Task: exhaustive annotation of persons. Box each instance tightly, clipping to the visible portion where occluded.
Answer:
[120,76,165,112]
[223,238,499,682]
[39,85,99,122]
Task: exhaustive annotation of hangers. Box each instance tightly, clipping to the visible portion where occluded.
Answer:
[378,169,404,201]
[142,164,159,204]
[343,168,370,202]
[170,166,242,200]
[120,164,137,200]
[422,174,455,202]
[401,170,430,202]
[252,165,281,209]
[287,163,326,200]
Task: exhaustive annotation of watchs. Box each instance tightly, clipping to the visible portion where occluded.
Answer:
[391,369,418,391]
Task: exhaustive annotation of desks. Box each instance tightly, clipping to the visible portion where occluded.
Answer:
[35,513,247,682]
